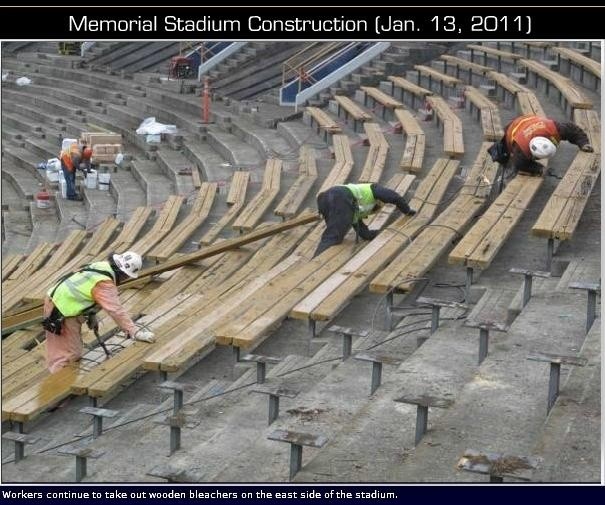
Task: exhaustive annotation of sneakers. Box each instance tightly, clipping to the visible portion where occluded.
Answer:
[70,196,82,200]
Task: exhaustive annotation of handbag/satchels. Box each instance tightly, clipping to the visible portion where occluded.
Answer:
[487,143,509,164]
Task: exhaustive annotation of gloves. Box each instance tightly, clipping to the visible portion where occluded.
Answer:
[539,168,553,177]
[88,171,92,173]
[135,331,155,343]
[582,144,593,152]
[84,171,86,178]
[406,210,415,215]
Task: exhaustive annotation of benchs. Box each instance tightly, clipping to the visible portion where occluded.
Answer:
[2,41,601,434]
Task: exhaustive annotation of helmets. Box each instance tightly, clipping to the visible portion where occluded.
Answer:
[113,252,142,278]
[84,148,92,160]
[528,136,556,159]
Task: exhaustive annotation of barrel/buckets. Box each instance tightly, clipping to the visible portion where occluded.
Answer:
[98,173,110,190]
[83,169,97,188]
[36,192,51,208]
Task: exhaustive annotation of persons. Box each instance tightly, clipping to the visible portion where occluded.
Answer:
[499,113,593,188]
[42,250,157,375]
[61,143,92,201]
[313,181,418,258]
